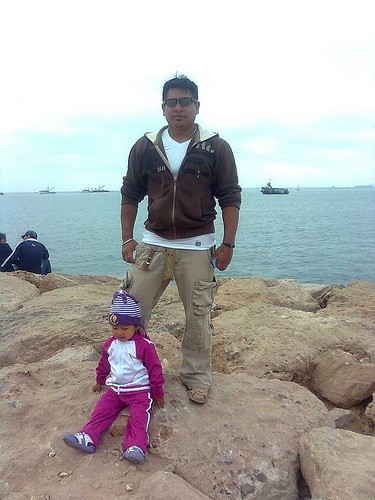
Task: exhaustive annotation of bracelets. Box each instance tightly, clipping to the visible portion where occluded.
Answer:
[122,238,134,246]
[222,243,235,248]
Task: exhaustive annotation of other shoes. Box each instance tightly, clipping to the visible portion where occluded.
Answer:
[187,384,209,404]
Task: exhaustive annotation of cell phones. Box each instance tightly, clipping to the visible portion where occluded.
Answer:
[211,259,218,268]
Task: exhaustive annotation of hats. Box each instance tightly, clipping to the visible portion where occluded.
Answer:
[109,290,143,326]
[21,230,38,240]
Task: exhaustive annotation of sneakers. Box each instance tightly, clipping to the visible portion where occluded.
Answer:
[63,432,96,454]
[122,446,145,464]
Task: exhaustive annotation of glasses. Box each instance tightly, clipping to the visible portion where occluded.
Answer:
[162,98,199,108]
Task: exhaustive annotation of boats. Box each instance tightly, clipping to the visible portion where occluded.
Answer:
[80,185,110,193]
[260,182,289,194]
[39,185,56,194]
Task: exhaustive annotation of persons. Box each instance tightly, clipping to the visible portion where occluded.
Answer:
[0,230,52,275]
[62,290,165,464]
[119,76,243,405]
